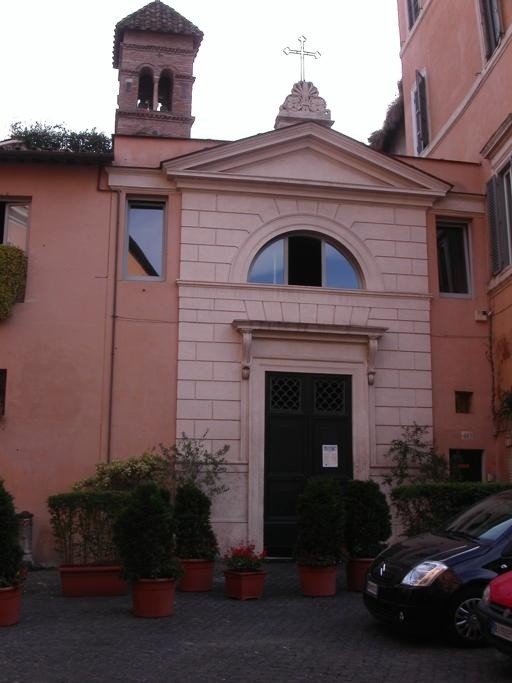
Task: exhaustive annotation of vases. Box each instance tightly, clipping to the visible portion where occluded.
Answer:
[224,567,267,599]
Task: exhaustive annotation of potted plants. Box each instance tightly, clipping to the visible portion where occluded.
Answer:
[293,470,392,597]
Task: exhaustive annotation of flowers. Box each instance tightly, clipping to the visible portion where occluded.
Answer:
[222,539,267,571]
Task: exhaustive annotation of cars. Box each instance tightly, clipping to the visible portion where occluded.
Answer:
[476,567,511,661]
[364,486,511,647]
